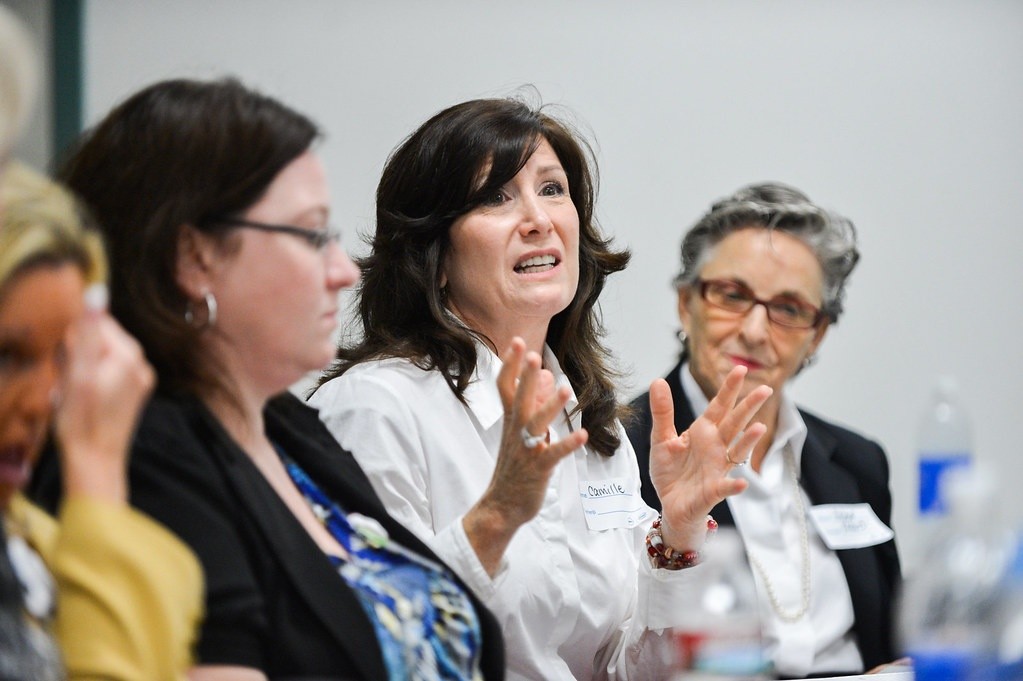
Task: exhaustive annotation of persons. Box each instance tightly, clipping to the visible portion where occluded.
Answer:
[1,77,903,681]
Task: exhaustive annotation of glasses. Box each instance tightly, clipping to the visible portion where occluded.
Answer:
[687,276,825,330]
[223,217,337,251]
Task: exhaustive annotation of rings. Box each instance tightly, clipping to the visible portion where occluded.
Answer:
[521,427,546,447]
[726,447,747,466]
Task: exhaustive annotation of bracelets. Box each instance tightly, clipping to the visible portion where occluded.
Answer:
[646,513,718,569]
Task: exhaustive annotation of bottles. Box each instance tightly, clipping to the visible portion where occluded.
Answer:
[671,528,776,681]
[899,372,1023,681]
